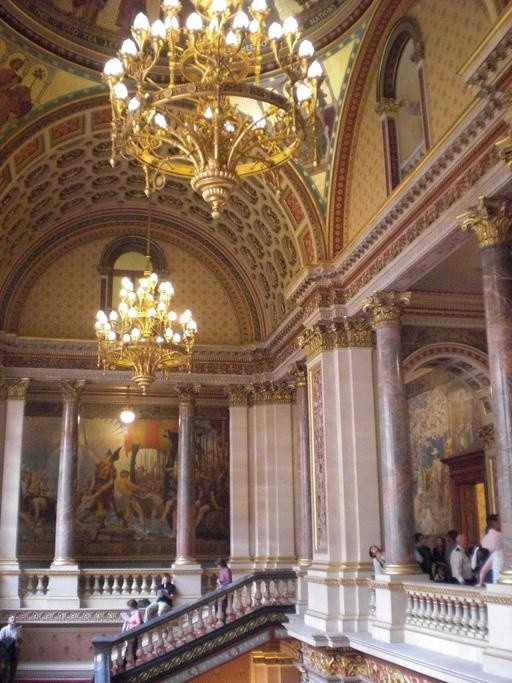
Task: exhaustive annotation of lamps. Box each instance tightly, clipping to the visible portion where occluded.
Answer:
[120,387,136,424]
[95,200,199,394]
[99,0,323,220]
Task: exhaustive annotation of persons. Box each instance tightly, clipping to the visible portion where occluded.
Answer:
[416,514,502,587]
[0,615,24,683]
[143,574,176,623]
[122,599,142,633]
[369,546,385,568]
[214,558,232,624]
[20,439,228,543]
